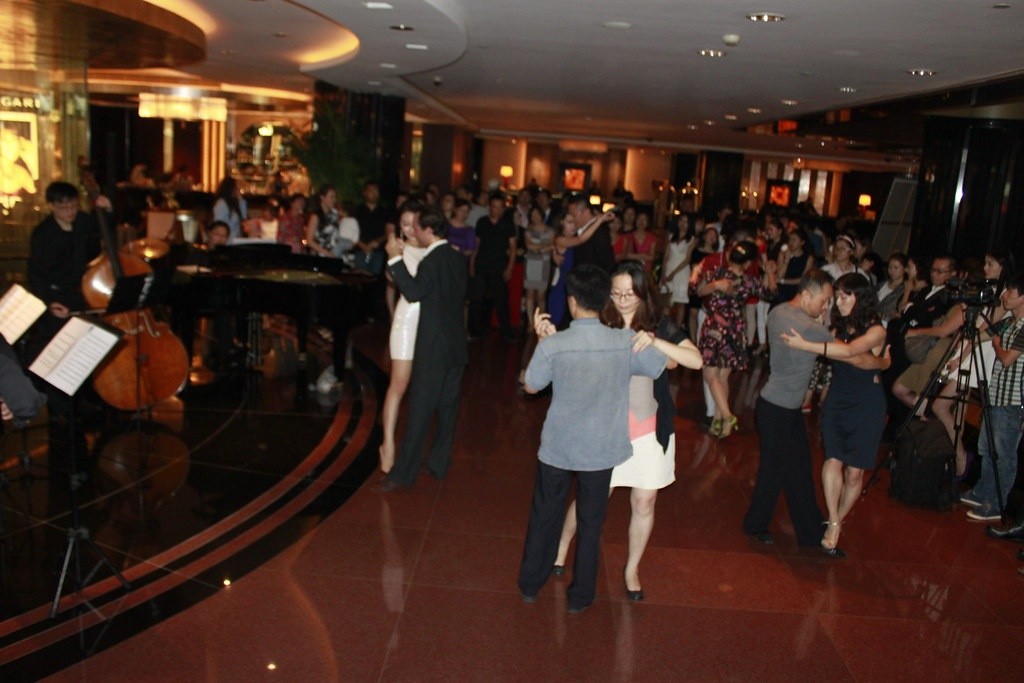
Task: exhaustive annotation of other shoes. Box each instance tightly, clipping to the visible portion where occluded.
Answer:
[801,404,811,412]
[371,478,413,492]
[966,506,1002,519]
[743,529,773,544]
[568,603,586,614]
[522,593,538,602]
[814,544,847,558]
[421,463,439,478]
[959,491,983,506]
[501,331,518,343]
[904,335,936,363]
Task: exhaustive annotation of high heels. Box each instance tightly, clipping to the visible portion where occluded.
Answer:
[709,418,722,436]
[552,564,565,575]
[719,416,738,438]
[955,452,977,481]
[623,565,643,601]
[379,445,394,472]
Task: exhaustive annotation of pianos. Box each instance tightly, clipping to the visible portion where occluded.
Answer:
[155,236,385,388]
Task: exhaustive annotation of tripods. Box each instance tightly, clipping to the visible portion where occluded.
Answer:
[861,299,1009,531]
[0,282,133,620]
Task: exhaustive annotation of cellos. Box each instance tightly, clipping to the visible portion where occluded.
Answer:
[78,166,188,410]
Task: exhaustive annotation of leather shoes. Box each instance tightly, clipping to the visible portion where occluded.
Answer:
[987,520,1024,541]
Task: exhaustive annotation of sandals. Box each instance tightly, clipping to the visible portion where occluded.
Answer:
[821,522,841,549]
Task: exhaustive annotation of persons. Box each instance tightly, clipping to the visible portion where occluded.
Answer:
[28,181,116,484]
[116,161,1024,613]
[0,336,46,428]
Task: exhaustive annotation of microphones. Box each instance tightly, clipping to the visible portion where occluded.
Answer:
[946,280,967,290]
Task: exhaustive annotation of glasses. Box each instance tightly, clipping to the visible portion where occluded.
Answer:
[608,290,636,300]
[930,268,950,275]
[833,245,850,253]
[53,203,80,212]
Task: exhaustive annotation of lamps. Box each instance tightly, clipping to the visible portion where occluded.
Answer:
[500,165,513,187]
[858,195,872,215]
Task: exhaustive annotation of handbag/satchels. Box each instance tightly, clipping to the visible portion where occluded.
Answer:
[894,419,954,497]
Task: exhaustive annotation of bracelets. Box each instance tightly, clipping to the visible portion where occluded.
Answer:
[824,342,827,356]
